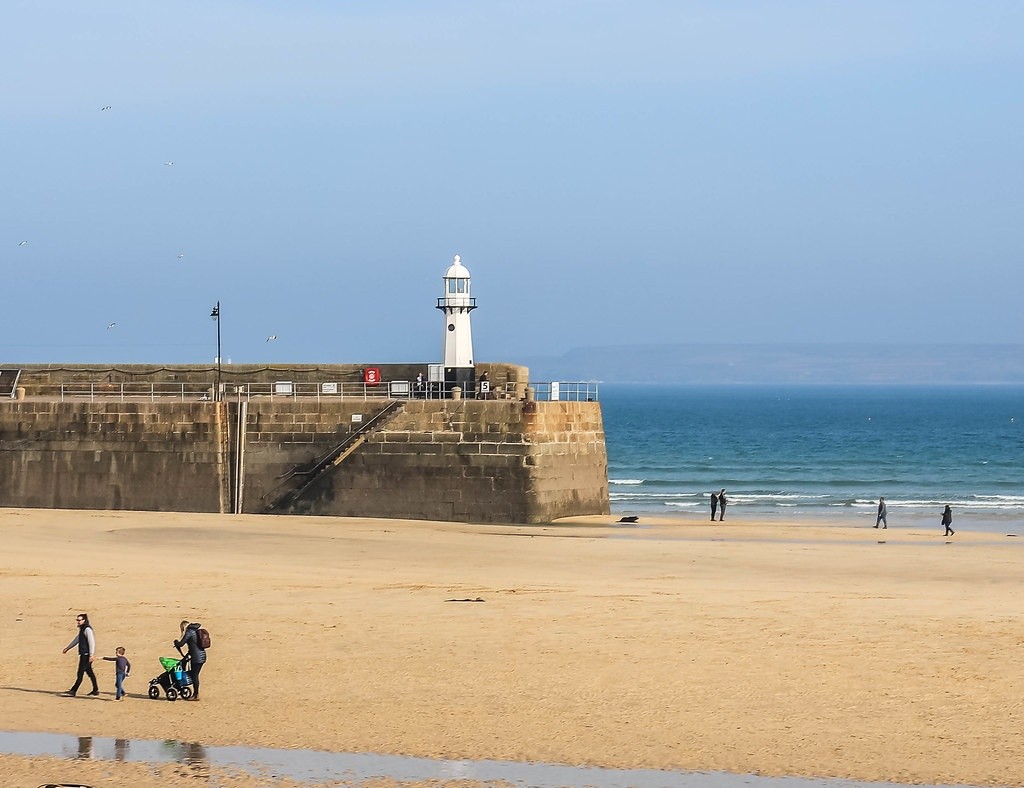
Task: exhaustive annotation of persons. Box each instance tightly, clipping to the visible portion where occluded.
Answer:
[173,620,211,701]
[719,489,727,521]
[710,491,718,522]
[97,647,131,702]
[60,613,99,697]
[940,504,955,536]
[873,497,888,529]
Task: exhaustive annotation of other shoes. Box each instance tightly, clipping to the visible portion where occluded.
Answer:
[59,691,75,697]
[122,693,128,701]
[114,696,121,702]
[187,693,199,701]
[86,690,99,696]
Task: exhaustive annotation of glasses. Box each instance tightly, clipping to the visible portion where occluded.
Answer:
[76,619,84,622]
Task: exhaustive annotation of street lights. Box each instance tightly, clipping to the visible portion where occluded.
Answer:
[209,301,221,400]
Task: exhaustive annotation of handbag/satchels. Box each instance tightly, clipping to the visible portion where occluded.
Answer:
[177,670,194,687]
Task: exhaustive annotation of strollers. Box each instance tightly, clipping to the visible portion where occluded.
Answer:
[148,652,192,701]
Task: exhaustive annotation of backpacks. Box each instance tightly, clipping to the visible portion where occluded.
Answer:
[196,628,211,649]
